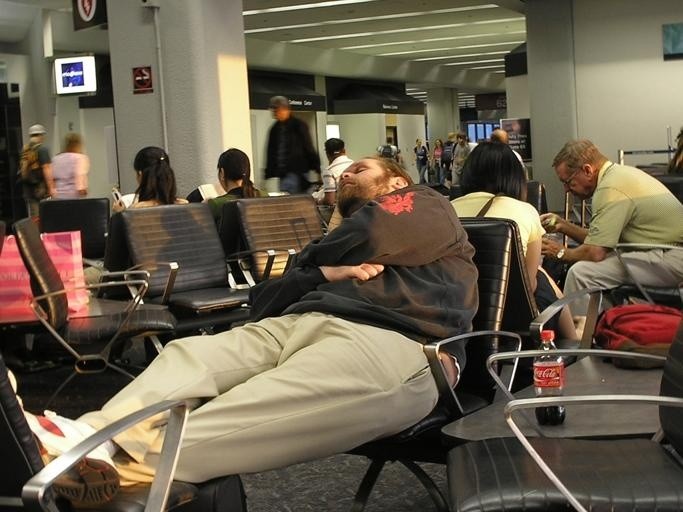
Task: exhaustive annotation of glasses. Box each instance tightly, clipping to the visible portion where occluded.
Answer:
[560,169,580,185]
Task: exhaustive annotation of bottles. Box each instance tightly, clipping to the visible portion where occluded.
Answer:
[532,330,565,425]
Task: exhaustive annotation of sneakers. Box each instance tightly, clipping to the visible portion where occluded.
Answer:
[14,409,120,508]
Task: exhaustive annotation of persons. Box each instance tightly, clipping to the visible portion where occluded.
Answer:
[15,124,90,220]
[263,96,322,195]
[449,141,578,339]
[206,149,269,224]
[21,156,480,507]
[112,146,189,213]
[315,138,353,208]
[539,139,683,314]
[381,136,398,158]
[415,131,472,188]
[490,130,525,168]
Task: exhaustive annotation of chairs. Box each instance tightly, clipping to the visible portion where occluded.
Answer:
[2,192,339,408]
[347,148,682,512]
[0,358,247,511]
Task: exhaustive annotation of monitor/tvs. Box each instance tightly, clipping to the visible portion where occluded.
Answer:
[51,51,97,97]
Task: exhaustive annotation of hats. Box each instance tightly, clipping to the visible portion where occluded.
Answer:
[28,124,48,134]
[325,138,344,155]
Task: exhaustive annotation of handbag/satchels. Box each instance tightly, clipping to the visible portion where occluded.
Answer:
[0,230,90,323]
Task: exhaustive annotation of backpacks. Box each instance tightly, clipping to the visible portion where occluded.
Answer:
[16,143,42,186]
[590,303,682,370]
[381,146,392,157]
